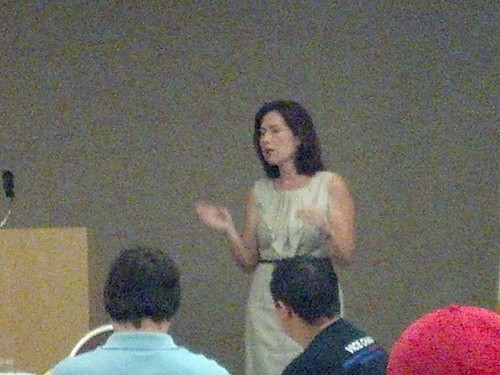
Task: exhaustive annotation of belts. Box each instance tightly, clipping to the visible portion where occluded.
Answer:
[257,258,277,263]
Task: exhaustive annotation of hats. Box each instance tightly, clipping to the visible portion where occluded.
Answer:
[387,303,500,375]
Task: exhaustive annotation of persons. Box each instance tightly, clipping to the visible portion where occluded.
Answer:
[195,99,355,375]
[269,256,390,375]
[51,244,233,375]
[387,304,500,375]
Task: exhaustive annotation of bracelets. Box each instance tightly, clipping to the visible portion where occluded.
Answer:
[320,228,334,243]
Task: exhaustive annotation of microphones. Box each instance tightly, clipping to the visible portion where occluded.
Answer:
[2,170,15,197]
